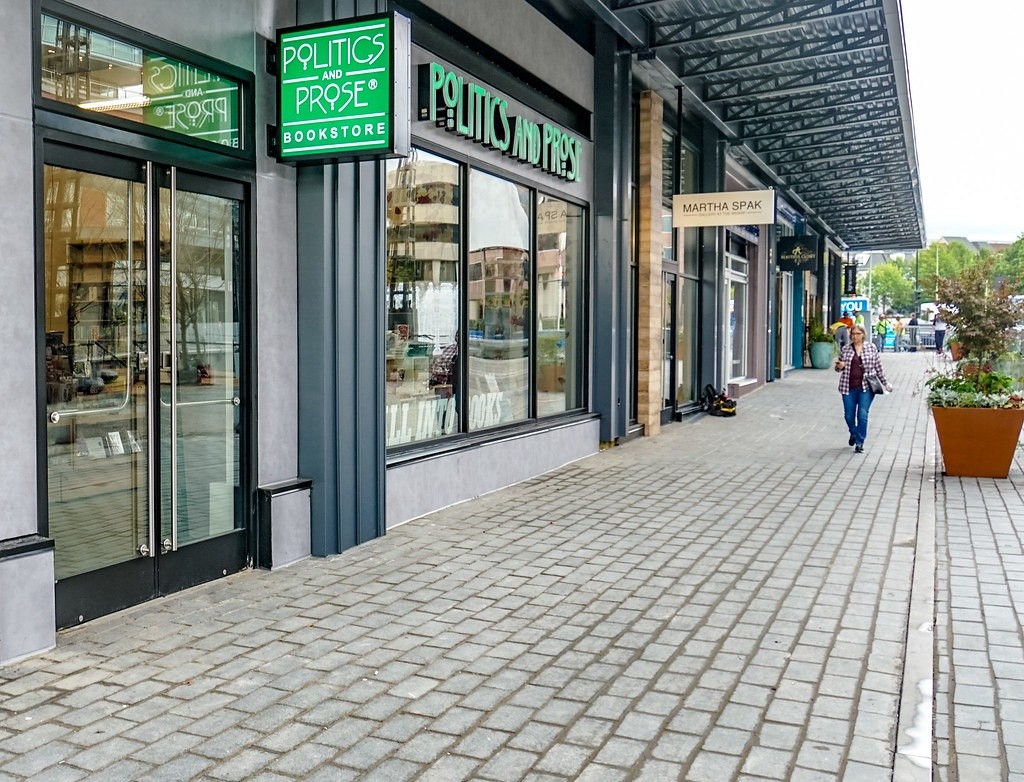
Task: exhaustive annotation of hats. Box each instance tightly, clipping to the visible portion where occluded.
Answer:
[843,311,850,316]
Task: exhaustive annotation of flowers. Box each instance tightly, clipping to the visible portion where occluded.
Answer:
[922,366,1024,410]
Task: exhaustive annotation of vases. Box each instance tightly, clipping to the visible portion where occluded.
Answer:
[931,406,1023,478]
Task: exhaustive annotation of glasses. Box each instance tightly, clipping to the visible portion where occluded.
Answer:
[851,333,862,336]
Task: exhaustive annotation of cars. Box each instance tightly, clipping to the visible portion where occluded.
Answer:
[58,358,118,395]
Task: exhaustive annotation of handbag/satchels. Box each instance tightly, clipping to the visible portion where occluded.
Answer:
[866,376,884,395]
[705,383,737,417]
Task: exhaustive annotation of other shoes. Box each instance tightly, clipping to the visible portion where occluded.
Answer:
[856,446,864,452]
[849,436,856,446]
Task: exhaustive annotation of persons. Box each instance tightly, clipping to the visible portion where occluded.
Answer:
[892,315,905,352]
[932,306,950,356]
[874,313,890,353]
[907,312,918,352]
[829,310,864,352]
[835,323,893,452]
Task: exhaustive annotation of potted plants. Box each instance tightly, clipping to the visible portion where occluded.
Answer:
[536,336,568,394]
[805,309,839,369]
